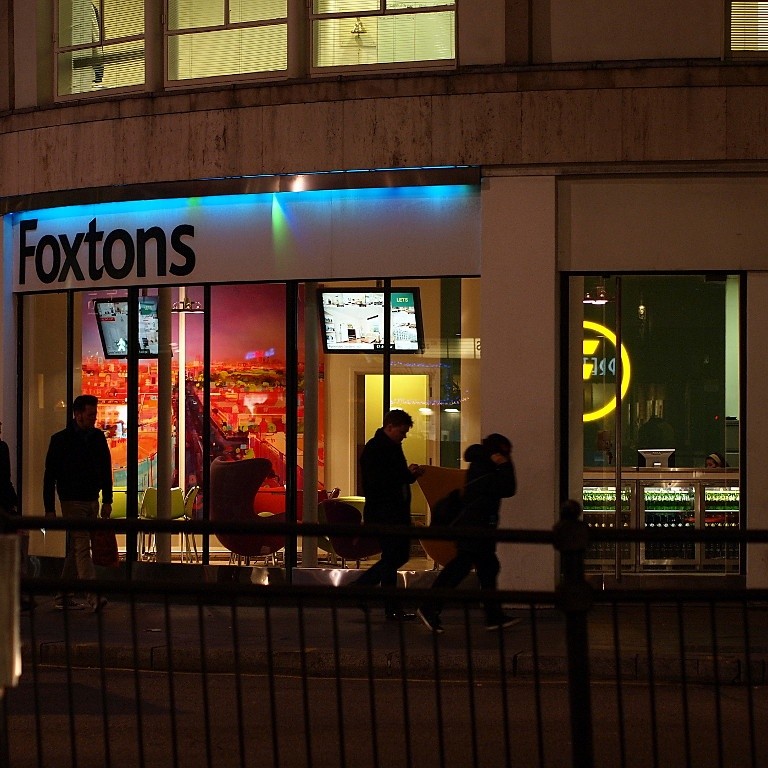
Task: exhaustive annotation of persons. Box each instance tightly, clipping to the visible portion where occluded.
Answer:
[352,409,425,621]
[416,433,522,633]
[42,396,113,612]
[706,453,730,468]
[0,441,38,613]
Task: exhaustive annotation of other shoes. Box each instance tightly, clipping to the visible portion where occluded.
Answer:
[486,614,520,630]
[346,581,370,614]
[386,609,416,621]
[54,595,86,610]
[88,595,108,613]
[418,605,444,634]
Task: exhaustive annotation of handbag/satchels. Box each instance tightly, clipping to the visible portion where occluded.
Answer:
[90,527,120,567]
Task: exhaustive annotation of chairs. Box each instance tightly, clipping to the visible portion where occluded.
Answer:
[98,453,469,570]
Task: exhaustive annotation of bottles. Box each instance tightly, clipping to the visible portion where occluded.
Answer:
[645,541,695,560]
[582,491,630,511]
[704,493,740,510]
[583,542,630,559]
[644,493,693,510]
[704,543,738,559]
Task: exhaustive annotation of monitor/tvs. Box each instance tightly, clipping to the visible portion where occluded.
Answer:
[316,287,425,355]
[93,295,159,359]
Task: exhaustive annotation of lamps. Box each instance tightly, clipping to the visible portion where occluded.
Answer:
[581,275,617,305]
